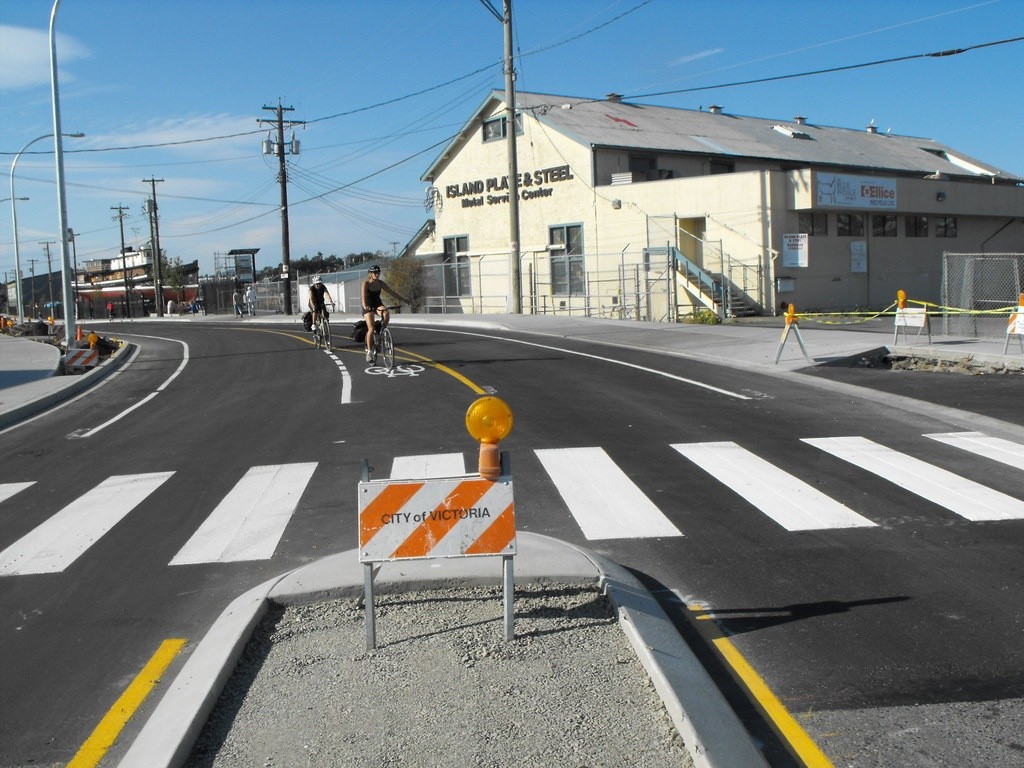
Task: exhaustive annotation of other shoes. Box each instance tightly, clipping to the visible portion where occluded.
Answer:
[366,352,373,363]
[311,324,317,331]
[241,314,243,318]
[380,341,390,349]
[253,312,256,316]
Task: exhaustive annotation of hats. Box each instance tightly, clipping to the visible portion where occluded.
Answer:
[247,286,251,290]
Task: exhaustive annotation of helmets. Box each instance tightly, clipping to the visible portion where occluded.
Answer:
[313,276,323,283]
[369,265,381,272]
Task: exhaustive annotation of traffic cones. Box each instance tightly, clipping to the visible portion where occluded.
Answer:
[77,325,83,342]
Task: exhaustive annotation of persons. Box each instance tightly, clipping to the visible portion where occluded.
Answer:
[167,297,175,314]
[192,300,199,315]
[107,302,113,318]
[245,287,257,316]
[308,275,335,330]
[361,264,414,361]
[232,289,243,318]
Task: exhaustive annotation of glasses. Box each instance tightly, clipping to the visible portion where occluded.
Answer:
[316,283,322,285]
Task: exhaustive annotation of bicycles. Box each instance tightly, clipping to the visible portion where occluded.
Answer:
[363,305,402,373]
[311,303,335,351]
[107,310,115,324]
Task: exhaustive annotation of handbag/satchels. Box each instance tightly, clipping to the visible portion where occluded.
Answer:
[351,321,368,344]
[303,312,313,332]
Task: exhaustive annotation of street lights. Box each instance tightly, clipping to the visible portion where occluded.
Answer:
[10,131,86,323]
[1,197,30,202]
